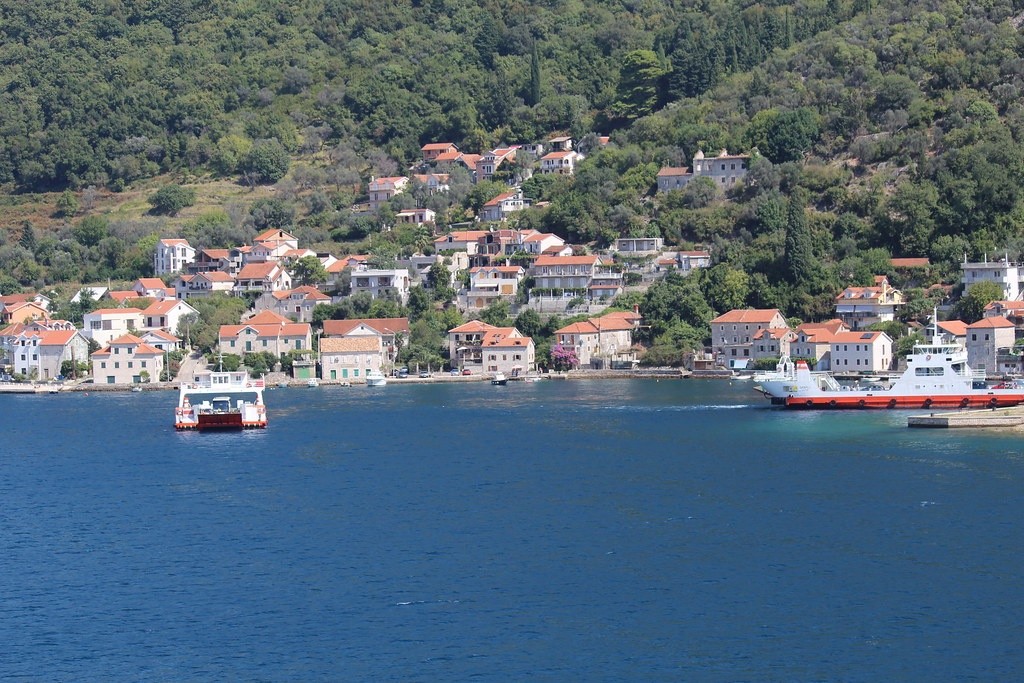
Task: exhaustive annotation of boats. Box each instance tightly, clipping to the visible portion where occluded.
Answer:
[752,305,1024,410]
[491,371,509,385]
[366,368,386,386]
[175,332,268,430]
[308,378,319,387]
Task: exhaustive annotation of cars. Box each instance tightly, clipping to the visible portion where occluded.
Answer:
[990,379,1018,389]
[398,372,408,379]
[462,369,470,375]
[418,371,429,378]
[450,369,460,376]
[860,385,885,391]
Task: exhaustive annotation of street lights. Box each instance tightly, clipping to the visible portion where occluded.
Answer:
[384,328,407,376]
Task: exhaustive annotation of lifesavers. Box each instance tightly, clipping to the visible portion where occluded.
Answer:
[830,399,837,406]
[806,400,813,406]
[962,398,969,404]
[991,397,998,403]
[890,399,897,405]
[925,398,932,405]
[858,399,866,405]
